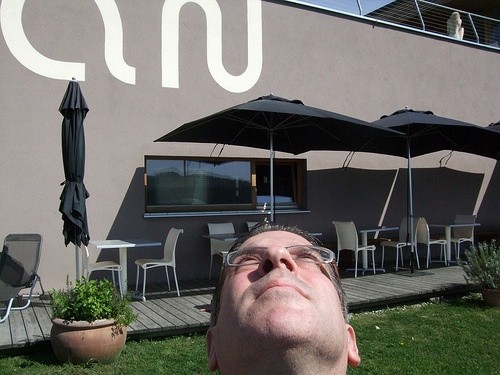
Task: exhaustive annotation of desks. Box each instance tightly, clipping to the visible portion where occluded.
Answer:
[203,232,322,243]
[428,222,481,266]
[89,239,163,297]
[346,225,399,275]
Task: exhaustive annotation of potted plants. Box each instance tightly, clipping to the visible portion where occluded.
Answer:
[457,238,500,305]
[48,273,139,365]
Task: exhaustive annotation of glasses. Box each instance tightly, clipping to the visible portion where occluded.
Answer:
[213,245,348,326]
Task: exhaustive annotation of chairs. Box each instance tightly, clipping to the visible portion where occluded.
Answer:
[80,241,124,300]
[135,228,184,302]
[332,220,377,278]
[381,214,476,270]
[207,222,240,280]
[0,233,44,324]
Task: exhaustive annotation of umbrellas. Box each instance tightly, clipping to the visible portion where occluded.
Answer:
[153,94,404,224]
[369,106,500,273]
[58,76,90,283]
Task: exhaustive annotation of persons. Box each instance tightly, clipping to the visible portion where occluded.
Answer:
[205,224,359,375]
[447,12,462,40]
